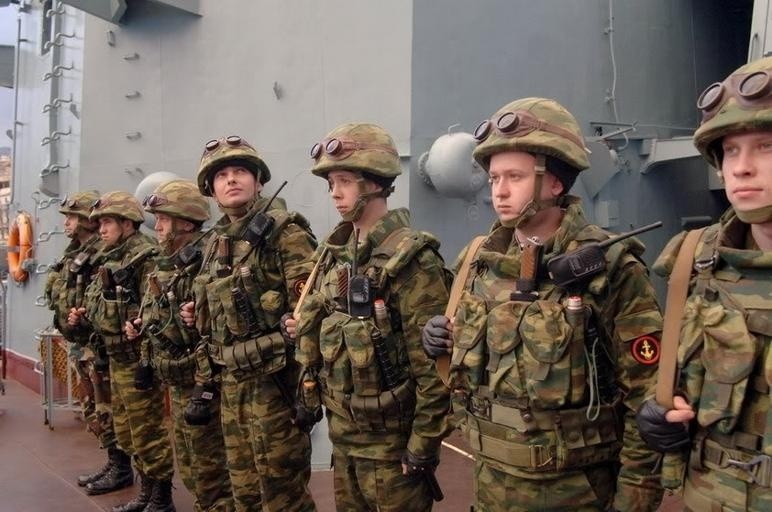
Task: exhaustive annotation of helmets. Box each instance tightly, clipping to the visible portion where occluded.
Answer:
[472,97,590,176]
[143,179,212,222]
[310,121,402,179]
[87,190,145,224]
[196,134,271,197]
[692,55,772,169]
[58,193,100,218]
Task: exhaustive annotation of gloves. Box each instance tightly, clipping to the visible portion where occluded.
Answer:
[289,404,323,430]
[183,386,212,426]
[178,301,187,328]
[636,391,690,454]
[280,312,296,345]
[400,449,441,483]
[421,315,453,359]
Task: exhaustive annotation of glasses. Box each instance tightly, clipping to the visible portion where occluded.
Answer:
[141,195,174,207]
[59,198,80,209]
[473,108,540,150]
[88,199,112,212]
[695,68,772,122]
[200,135,256,162]
[310,136,356,161]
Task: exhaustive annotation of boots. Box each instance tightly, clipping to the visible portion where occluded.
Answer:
[104,479,152,512]
[77,448,115,486]
[143,478,177,512]
[84,450,134,494]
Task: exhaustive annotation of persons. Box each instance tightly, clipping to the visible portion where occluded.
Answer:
[177,135,323,512]
[280,125,453,512]
[638,54,772,512]
[44,190,133,495]
[422,96,664,511]
[67,191,175,511]
[127,179,234,512]
